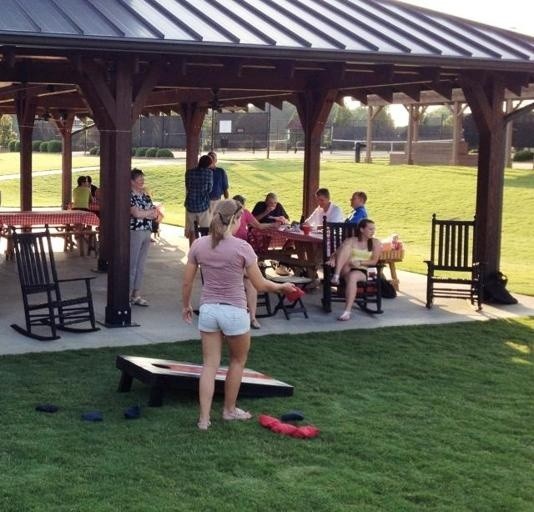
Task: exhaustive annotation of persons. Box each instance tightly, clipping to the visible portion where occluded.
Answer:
[342,192,367,240]
[73,176,98,212]
[182,199,298,430]
[130,167,164,307]
[326,220,382,321]
[185,155,213,248]
[208,151,230,216]
[302,189,343,288]
[249,193,289,234]
[231,195,281,330]
[88,177,101,196]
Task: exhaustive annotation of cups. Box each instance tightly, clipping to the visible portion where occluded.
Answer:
[303,225,311,236]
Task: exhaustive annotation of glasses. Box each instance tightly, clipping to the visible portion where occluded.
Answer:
[235,201,242,215]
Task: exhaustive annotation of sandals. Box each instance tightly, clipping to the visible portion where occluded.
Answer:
[223,407,252,421]
[336,310,352,320]
[199,417,211,431]
[129,299,133,308]
[252,318,262,329]
[132,296,150,306]
[331,273,340,284]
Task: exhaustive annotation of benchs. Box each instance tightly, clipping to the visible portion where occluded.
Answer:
[0,226,83,247]
[5,229,99,264]
[261,251,319,291]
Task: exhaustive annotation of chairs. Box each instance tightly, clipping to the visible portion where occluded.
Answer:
[194,221,210,286]
[321,216,385,312]
[425,213,484,311]
[11,222,104,338]
